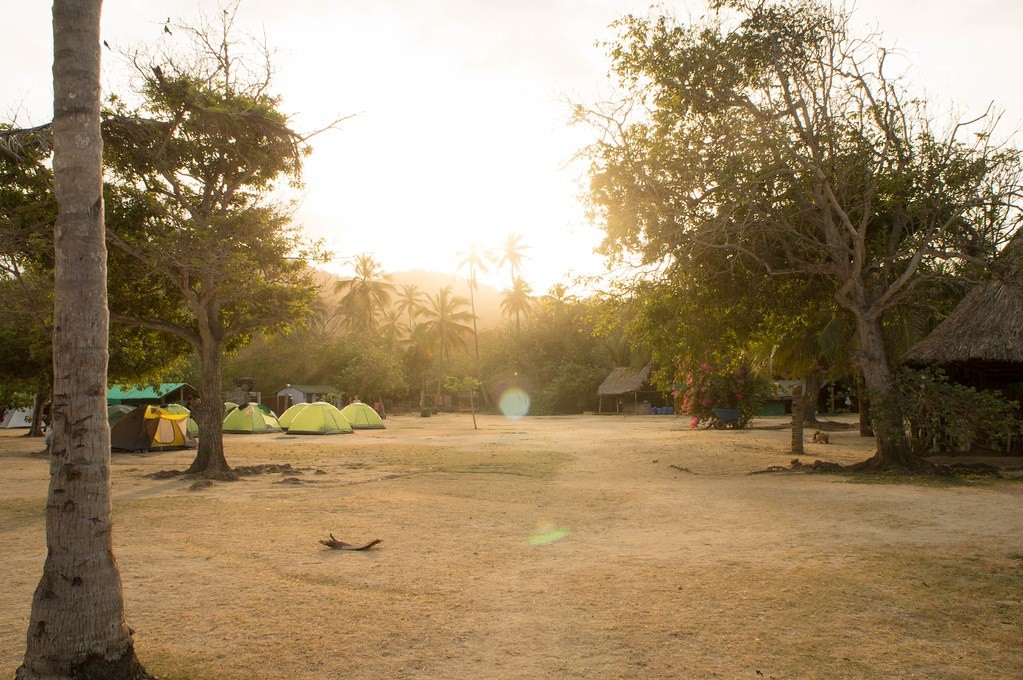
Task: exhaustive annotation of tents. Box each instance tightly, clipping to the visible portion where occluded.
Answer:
[285,402,353,436]
[276,402,312,428]
[156,402,202,437]
[107,405,199,451]
[220,401,239,424]
[220,402,283,435]
[0,406,47,428]
[105,405,137,432]
[339,403,385,429]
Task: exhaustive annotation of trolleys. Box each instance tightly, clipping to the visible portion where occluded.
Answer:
[705,405,739,430]
